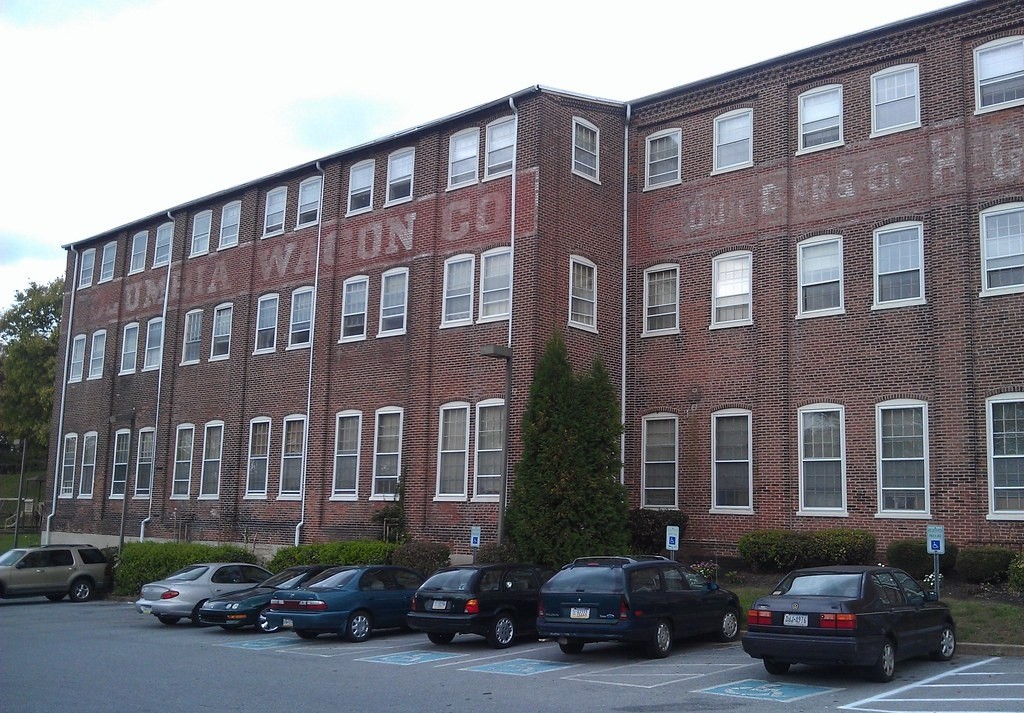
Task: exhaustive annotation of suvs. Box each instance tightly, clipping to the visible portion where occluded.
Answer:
[0,543,116,603]
[534,554,742,660]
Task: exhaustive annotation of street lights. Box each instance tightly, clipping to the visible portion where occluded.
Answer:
[480,344,513,548]
[12,437,28,548]
[108,410,137,556]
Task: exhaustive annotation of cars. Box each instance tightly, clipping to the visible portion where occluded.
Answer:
[742,567,957,685]
[135,563,274,628]
[266,565,421,643]
[407,562,559,650]
[197,564,336,634]
